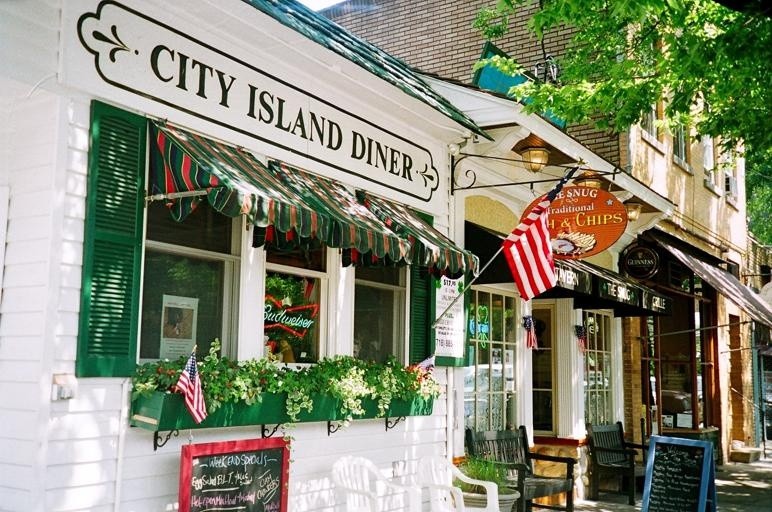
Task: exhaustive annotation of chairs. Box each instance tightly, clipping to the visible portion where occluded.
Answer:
[585,420,650,505]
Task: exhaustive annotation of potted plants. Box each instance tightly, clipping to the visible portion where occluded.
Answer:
[452,454,521,510]
[129,338,441,489]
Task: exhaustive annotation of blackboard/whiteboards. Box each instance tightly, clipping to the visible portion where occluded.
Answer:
[179,438,289,512]
[641,435,713,512]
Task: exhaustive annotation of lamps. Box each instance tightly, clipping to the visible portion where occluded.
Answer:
[518,147,551,174]
[572,177,603,189]
[623,201,643,222]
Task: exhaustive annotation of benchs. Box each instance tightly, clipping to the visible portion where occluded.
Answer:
[465,425,577,511]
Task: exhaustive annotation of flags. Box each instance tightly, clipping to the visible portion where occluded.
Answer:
[175,350,207,425]
[501,167,579,302]
[419,355,435,380]
